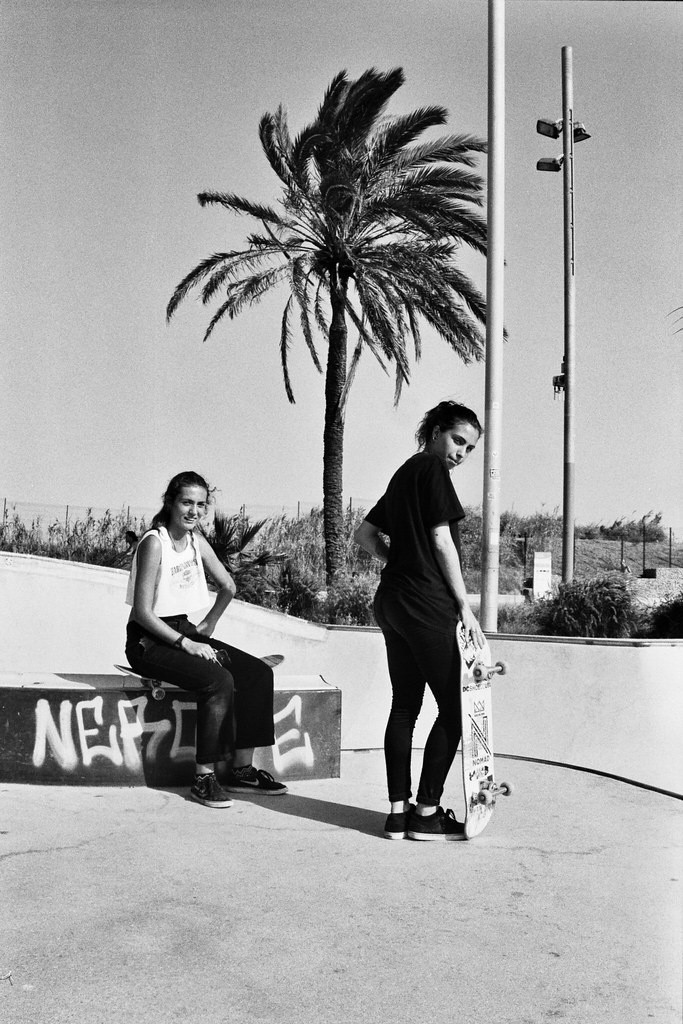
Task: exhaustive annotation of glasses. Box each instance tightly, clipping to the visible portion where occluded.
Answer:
[213,649,231,667]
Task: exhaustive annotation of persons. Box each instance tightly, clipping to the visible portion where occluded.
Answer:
[355,401,485,840]
[125,472,288,807]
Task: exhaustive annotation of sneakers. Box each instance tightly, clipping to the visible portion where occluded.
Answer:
[191,772,233,808]
[225,768,288,795]
[384,804,415,839]
[407,806,466,841]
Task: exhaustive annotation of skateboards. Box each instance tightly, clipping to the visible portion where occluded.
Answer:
[454,619,517,841]
[112,650,287,703]
